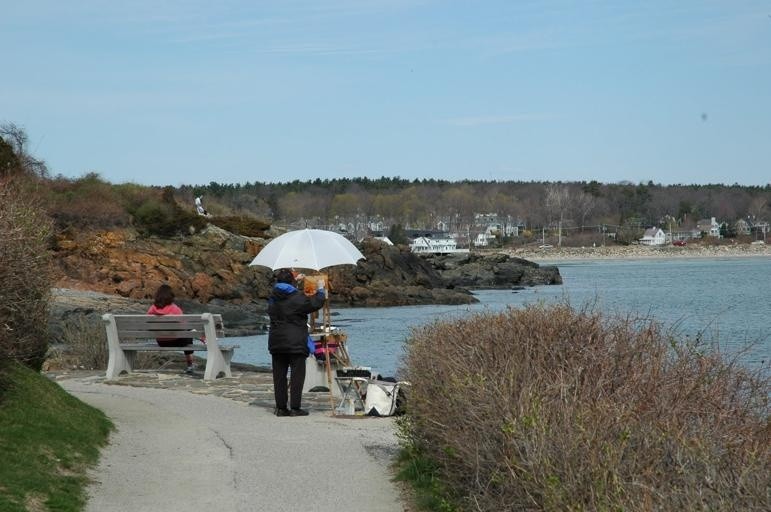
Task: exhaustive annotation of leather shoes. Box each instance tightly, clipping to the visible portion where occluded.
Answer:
[277,408,309,416]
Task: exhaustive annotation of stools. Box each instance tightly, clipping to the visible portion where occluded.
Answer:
[335,377,369,412]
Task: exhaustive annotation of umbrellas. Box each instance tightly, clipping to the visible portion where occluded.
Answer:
[247,226,368,273]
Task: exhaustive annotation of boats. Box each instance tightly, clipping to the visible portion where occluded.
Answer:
[308,330,344,361]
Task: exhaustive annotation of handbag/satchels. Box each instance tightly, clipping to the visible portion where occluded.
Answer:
[307,335,315,354]
[365,381,413,417]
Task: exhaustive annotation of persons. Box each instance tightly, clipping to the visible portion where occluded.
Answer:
[194,195,208,215]
[145,284,207,373]
[266,269,327,417]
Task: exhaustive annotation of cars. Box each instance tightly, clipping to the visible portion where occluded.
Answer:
[673,241,687,246]
[539,244,553,249]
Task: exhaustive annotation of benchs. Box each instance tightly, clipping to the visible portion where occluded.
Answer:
[102,313,241,381]
